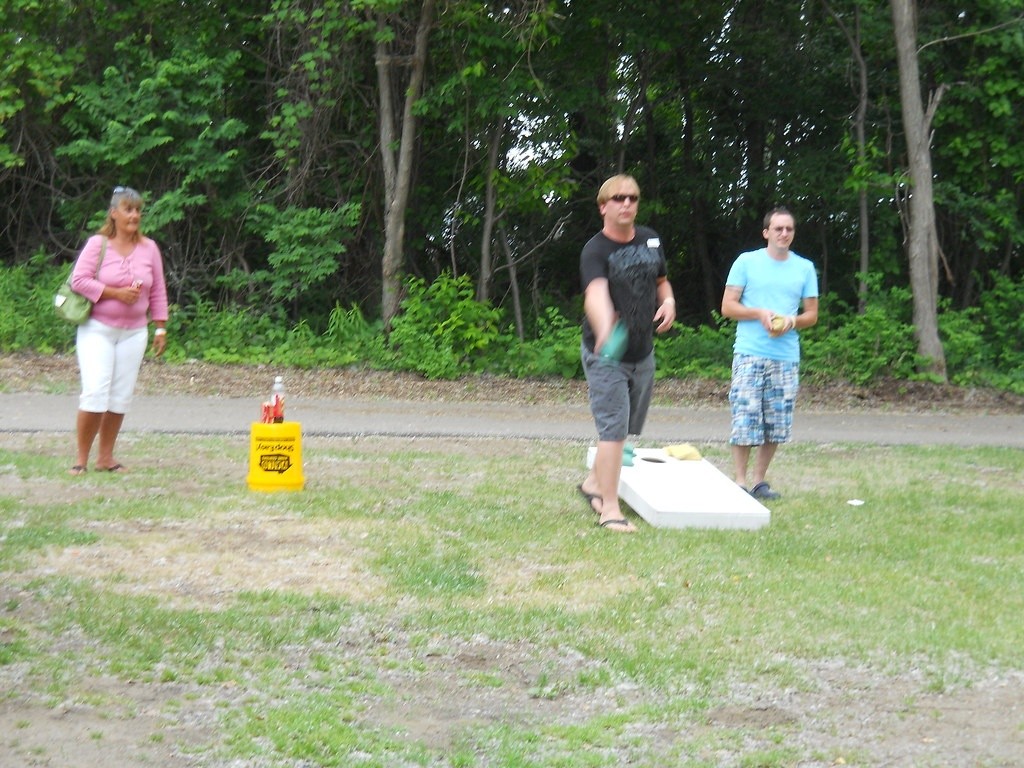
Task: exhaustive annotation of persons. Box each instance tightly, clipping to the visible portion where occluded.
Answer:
[68,186,169,475]
[722,208,818,500]
[581,174,676,533]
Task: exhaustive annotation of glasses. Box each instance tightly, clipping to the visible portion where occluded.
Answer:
[607,194,641,204]
[113,187,124,196]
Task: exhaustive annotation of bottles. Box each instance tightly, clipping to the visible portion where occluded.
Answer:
[271,376,284,422]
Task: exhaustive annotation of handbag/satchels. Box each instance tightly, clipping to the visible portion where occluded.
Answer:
[55,233,108,324]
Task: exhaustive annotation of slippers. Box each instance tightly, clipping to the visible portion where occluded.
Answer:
[95,462,121,473]
[579,485,604,515]
[594,518,629,529]
[740,485,748,492]
[69,466,87,477]
[750,481,780,499]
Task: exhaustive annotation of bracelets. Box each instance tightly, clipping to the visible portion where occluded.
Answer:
[155,328,167,336]
[790,316,796,329]
